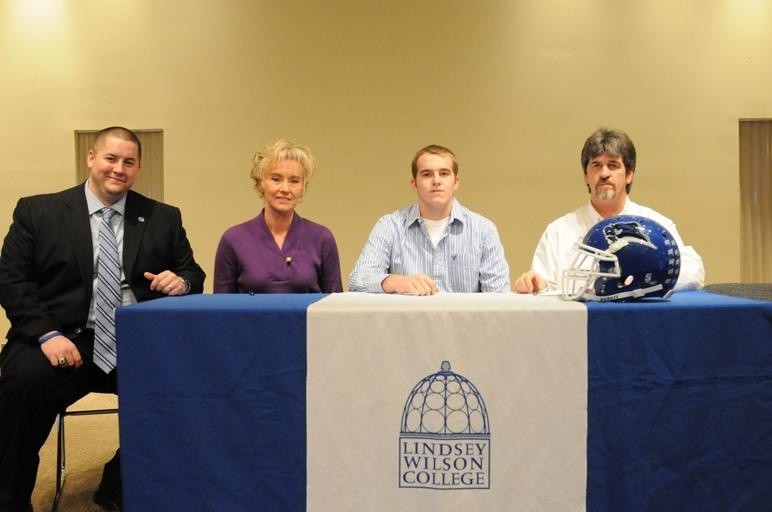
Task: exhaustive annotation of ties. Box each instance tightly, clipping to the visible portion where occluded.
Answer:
[93,207,122,376]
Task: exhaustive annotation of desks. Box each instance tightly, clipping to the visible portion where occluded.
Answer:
[114,291,772,512]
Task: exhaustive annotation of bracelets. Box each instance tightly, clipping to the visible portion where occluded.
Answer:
[38,331,64,345]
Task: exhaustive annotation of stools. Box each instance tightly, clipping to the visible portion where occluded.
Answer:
[51,407,119,512]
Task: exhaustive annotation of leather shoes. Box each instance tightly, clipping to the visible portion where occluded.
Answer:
[92,484,122,512]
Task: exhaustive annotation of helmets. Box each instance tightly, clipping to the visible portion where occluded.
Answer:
[562,215,680,303]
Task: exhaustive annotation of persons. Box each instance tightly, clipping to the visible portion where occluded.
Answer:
[211,136,345,295]
[0,126,206,511]
[346,144,512,297]
[511,125,707,296]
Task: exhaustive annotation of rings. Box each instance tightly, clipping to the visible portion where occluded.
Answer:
[59,357,66,365]
